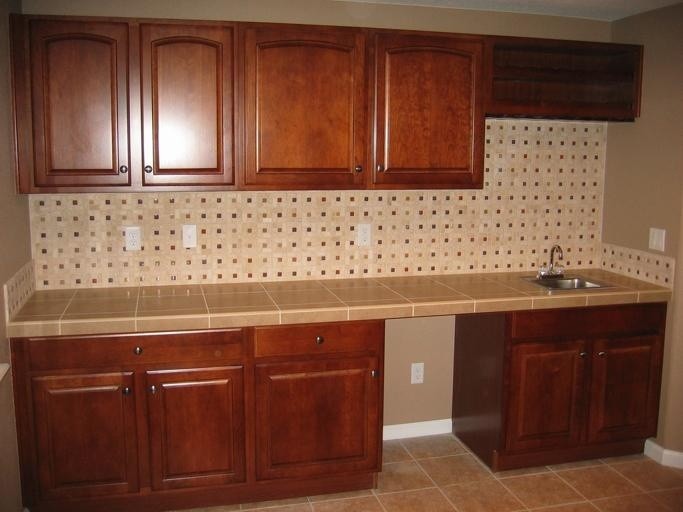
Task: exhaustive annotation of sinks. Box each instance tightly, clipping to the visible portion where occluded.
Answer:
[521,275,604,290]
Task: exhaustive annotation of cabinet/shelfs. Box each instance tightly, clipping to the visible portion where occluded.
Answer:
[452,303,667,472]
[252,321,383,505]
[481,34,646,122]
[371,27,482,188]
[10,14,133,195]
[240,21,368,192]
[132,18,239,190]
[7,326,252,512]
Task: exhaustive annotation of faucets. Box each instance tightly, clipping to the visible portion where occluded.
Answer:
[549,244,563,274]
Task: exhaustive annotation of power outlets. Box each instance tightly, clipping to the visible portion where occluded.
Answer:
[125,226,140,250]
[182,224,197,248]
[648,227,665,251]
[410,362,424,387]
[357,223,371,245]
